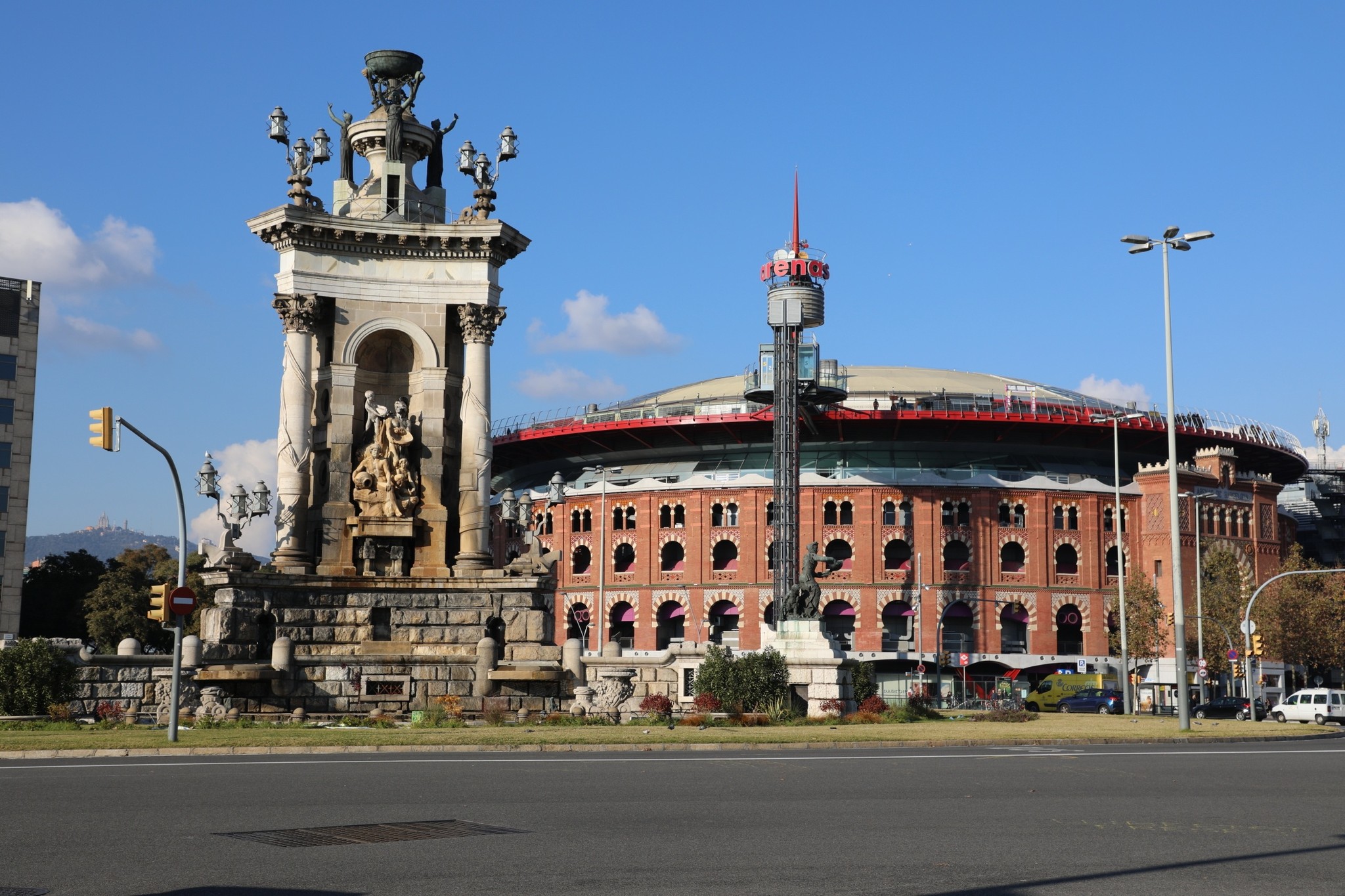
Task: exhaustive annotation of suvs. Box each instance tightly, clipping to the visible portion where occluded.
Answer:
[1271,689,1345,726]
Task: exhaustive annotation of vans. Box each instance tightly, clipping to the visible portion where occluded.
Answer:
[1025,674,1104,712]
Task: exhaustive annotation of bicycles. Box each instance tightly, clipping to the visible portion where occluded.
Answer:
[949,693,1015,711]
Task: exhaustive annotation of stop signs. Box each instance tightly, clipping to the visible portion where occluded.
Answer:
[169,587,196,615]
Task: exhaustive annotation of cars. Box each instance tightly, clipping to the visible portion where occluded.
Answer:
[1192,698,1267,721]
[1055,688,1125,715]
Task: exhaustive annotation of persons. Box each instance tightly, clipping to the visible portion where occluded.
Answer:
[1189,695,1196,712]
[1266,699,1272,715]
[946,692,952,709]
[997,689,1003,708]
[427,113,457,187]
[1205,697,1210,705]
[990,689,997,700]
[352,390,419,519]
[976,692,979,708]
[1257,696,1261,701]
[799,541,836,618]
[378,79,414,160]
[783,584,799,613]
[328,103,353,181]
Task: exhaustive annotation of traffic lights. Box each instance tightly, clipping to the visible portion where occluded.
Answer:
[944,651,951,665]
[88,406,114,452]
[1253,635,1264,656]
[1246,649,1254,658]
[1012,599,1021,614]
[147,583,170,622]
[1167,612,1174,627]
[939,655,946,666]
[1233,664,1240,678]
[1238,673,1245,678]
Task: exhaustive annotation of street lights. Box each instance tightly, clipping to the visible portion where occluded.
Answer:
[1177,491,1217,704]
[1120,224,1214,729]
[582,464,624,655]
[677,584,710,644]
[1089,413,1148,714]
[559,592,596,657]
